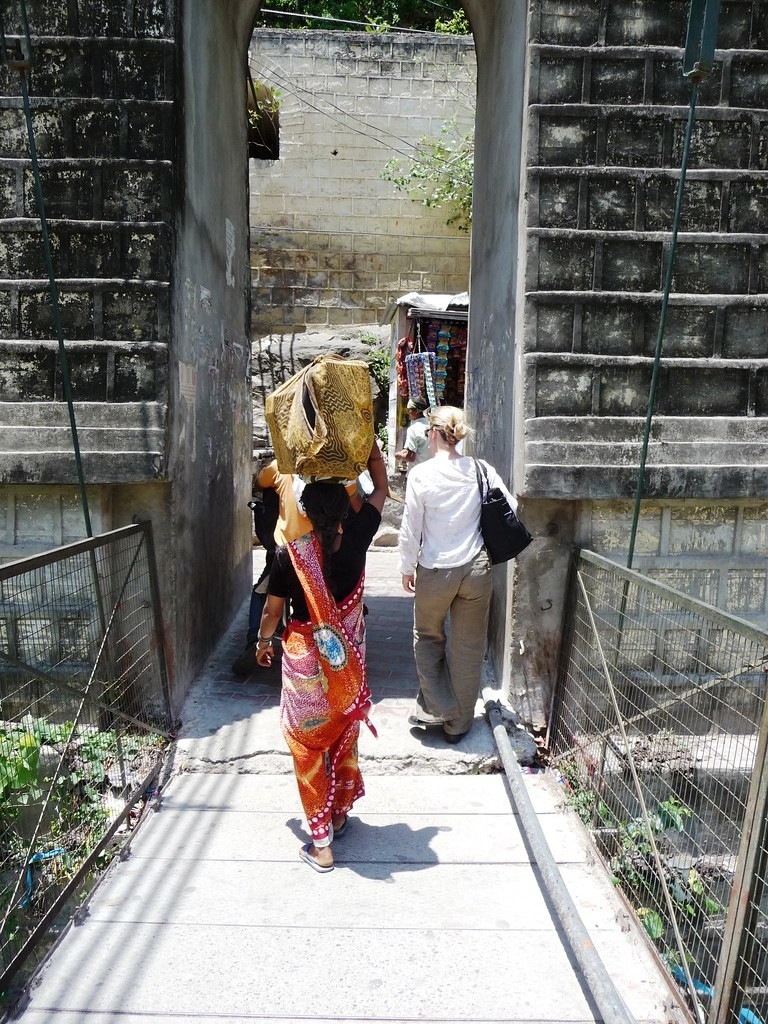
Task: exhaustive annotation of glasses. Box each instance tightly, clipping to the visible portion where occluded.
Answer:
[425,424,438,437]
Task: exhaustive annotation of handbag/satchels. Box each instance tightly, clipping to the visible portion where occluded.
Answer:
[474,459,533,565]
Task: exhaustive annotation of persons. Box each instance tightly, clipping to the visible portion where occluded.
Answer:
[232,438,389,873]
[398,405,519,745]
[393,396,434,478]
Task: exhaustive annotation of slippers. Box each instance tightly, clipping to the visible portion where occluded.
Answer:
[298,841,334,873]
[333,813,349,836]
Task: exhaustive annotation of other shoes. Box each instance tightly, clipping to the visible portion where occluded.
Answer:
[445,731,469,745]
[409,716,443,726]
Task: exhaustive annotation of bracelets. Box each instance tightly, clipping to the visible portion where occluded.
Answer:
[370,456,383,461]
[258,629,276,642]
[256,641,272,651]
